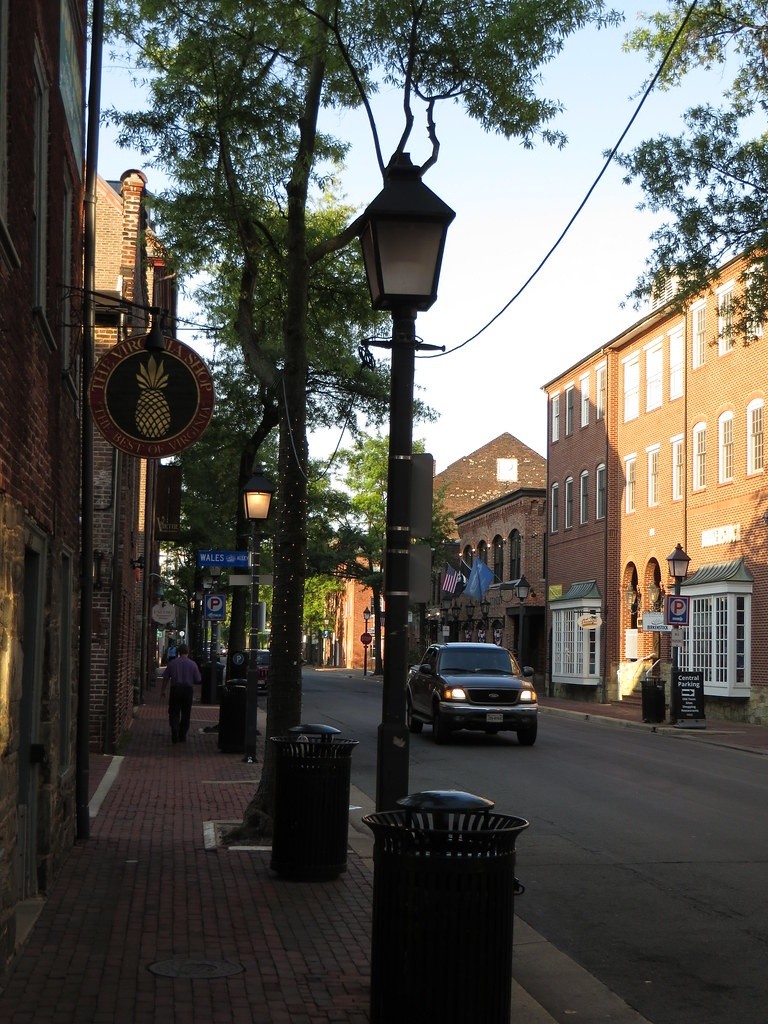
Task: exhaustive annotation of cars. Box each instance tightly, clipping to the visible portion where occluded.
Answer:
[206,640,227,654]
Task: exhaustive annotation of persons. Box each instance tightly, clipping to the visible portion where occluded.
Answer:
[161,638,178,667]
[160,642,202,745]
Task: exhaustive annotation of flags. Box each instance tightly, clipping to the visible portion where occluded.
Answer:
[442,559,471,598]
[464,556,495,603]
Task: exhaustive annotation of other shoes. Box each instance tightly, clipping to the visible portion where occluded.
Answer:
[171,737,187,746]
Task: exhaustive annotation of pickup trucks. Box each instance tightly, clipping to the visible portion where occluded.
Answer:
[405,640,540,749]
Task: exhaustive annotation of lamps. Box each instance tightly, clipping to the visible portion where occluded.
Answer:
[625,581,665,614]
[439,595,505,629]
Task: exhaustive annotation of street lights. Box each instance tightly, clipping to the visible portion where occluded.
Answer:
[238,465,277,762]
[665,544,691,727]
[516,574,528,670]
[186,593,204,680]
[348,150,454,1024]
[359,601,374,677]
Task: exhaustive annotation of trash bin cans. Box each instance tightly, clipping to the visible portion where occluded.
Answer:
[217,678,248,757]
[269,723,360,882]
[640,676,666,723]
[358,787,527,1024]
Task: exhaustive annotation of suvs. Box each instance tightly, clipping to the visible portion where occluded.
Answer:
[247,648,273,690]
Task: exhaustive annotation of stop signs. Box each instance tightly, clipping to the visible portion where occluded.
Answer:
[361,633,373,644]
